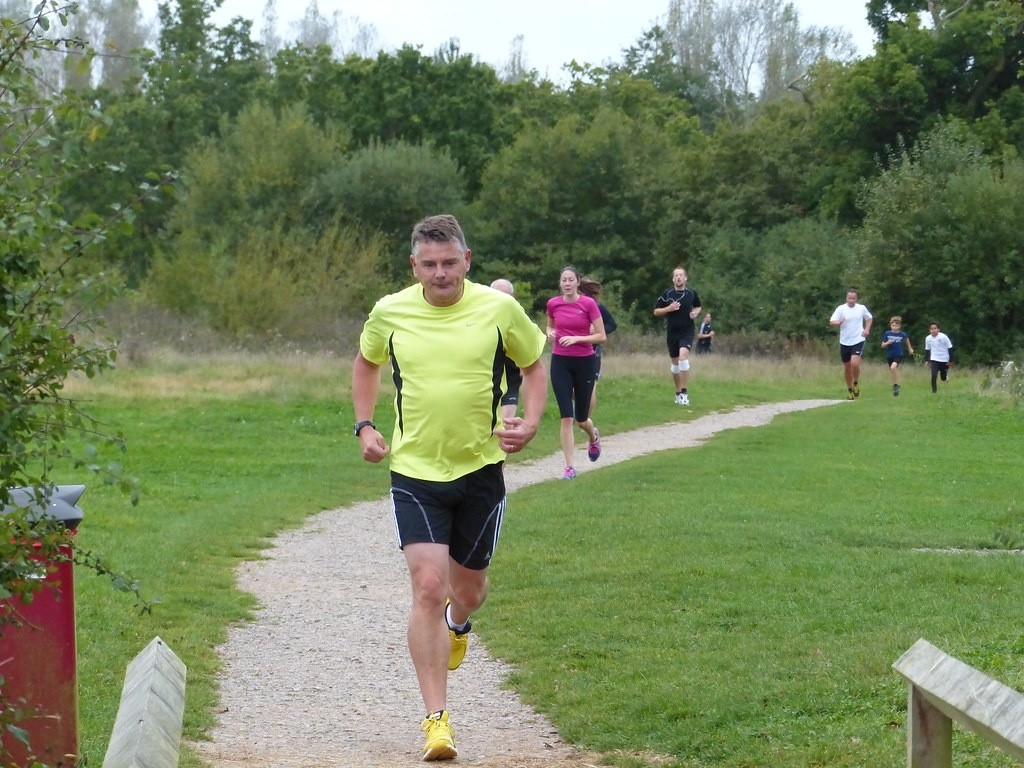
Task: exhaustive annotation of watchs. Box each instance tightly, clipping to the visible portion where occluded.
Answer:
[354,421,376,437]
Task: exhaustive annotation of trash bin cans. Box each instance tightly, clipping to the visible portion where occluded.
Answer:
[0,481,89,768]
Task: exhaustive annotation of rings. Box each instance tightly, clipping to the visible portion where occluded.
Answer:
[511,445,513,450]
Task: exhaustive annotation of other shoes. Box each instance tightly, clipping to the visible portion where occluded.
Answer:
[675,392,689,406]
[853,383,860,396]
[893,386,899,396]
[848,390,855,400]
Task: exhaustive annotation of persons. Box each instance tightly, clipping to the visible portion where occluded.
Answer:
[353,215,547,760]
[696,312,714,354]
[577,277,618,417]
[490,278,525,430]
[924,323,953,393]
[653,266,702,406]
[546,266,607,480]
[829,288,872,400]
[881,316,914,396]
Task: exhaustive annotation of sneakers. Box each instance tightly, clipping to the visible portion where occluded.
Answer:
[445,598,472,671]
[562,465,576,479]
[420,709,459,761]
[588,427,601,462]
